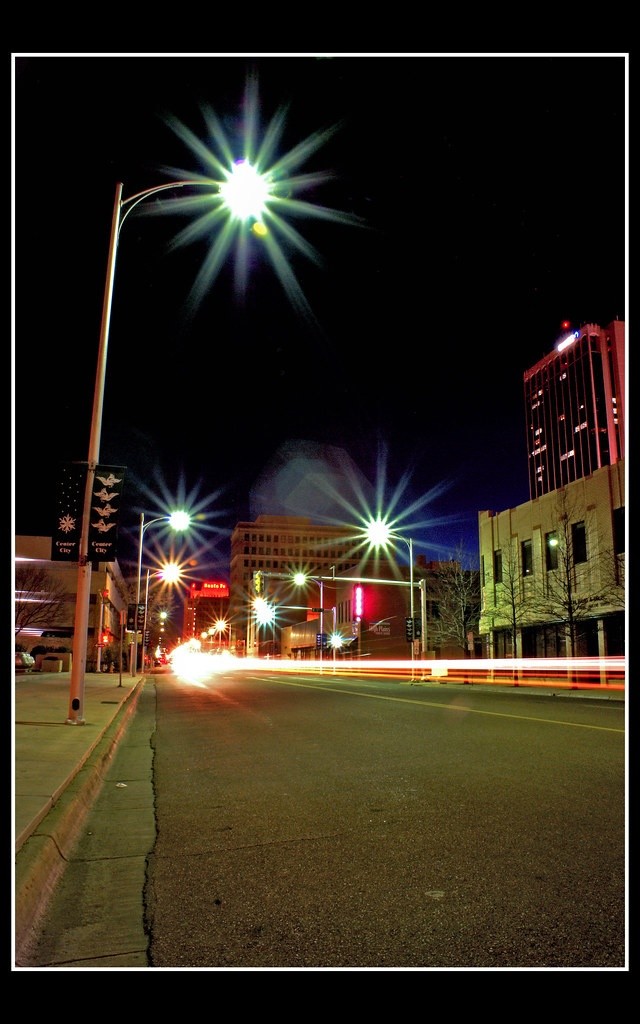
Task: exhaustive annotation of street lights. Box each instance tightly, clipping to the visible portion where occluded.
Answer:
[295,573,323,676]
[369,525,416,683]
[141,564,180,676]
[251,598,276,656]
[130,512,191,677]
[216,621,232,655]
[66,166,268,725]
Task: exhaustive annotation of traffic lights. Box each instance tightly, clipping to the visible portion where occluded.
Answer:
[351,584,366,620]
